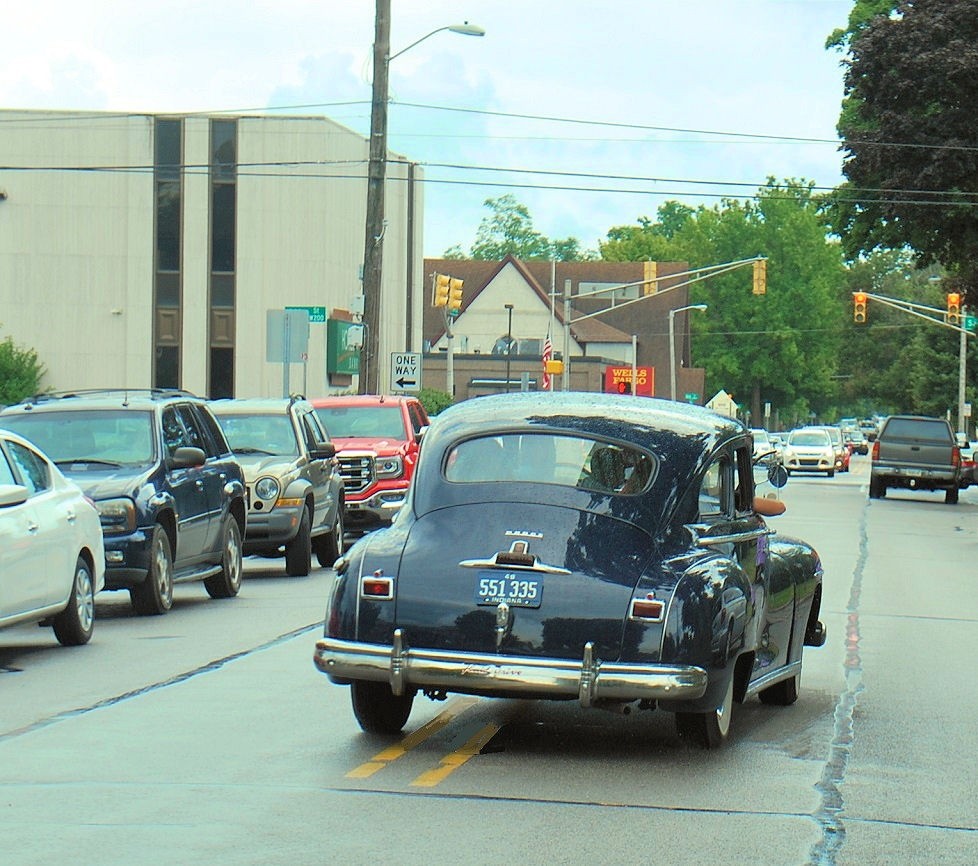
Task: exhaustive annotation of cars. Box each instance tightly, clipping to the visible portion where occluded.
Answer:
[0,428,106,648]
[733,414,978,490]
[309,388,834,749]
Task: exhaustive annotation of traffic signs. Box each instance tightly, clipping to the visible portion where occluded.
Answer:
[389,351,424,393]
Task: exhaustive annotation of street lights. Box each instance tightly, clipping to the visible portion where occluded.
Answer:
[504,304,515,392]
[667,304,708,401]
[356,20,488,395]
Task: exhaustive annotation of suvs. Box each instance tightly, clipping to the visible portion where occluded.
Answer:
[205,392,346,577]
[868,414,964,505]
[0,385,248,616]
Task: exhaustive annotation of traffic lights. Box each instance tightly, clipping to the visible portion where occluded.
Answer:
[433,273,451,308]
[947,293,961,326]
[447,277,465,310]
[853,294,867,325]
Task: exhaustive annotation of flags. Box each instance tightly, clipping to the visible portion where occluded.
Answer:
[543,323,554,391]
